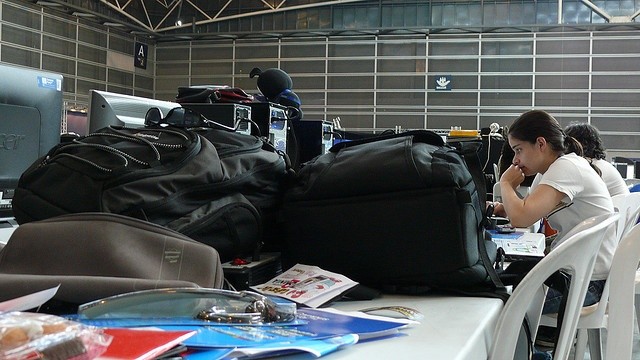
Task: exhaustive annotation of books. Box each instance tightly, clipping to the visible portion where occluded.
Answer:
[82,323,200,358]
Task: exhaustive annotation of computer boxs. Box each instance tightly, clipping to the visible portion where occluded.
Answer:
[254,107,288,152]
[297,122,334,160]
[182,100,251,137]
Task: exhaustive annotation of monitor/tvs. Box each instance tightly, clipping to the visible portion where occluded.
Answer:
[1,63,64,183]
[87,90,184,135]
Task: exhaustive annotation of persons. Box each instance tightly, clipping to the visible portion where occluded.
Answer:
[484,110,618,316]
[563,122,632,197]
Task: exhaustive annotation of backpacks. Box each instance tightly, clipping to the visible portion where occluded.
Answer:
[12,126,288,266]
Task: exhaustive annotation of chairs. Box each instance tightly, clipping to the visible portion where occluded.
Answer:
[545,221,639,360]
[488,213,620,359]
[611,192,640,238]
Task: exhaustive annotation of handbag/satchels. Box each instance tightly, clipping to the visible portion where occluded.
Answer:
[0,213,225,307]
[276,129,510,301]
[176,85,255,107]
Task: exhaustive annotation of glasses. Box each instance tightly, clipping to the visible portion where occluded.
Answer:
[144,107,242,131]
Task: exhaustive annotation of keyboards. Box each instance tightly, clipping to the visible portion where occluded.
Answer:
[0,202,15,218]
